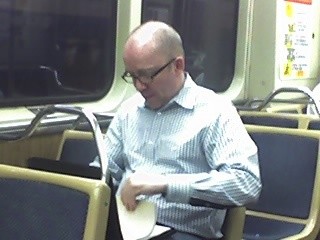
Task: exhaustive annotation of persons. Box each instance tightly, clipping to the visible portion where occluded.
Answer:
[86,18,262,240]
[306,82,320,116]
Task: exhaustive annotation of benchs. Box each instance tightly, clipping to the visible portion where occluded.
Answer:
[237,86,320,130]
[1,86,112,240]
[26,109,320,240]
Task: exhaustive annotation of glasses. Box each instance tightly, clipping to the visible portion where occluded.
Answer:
[121,58,177,86]
